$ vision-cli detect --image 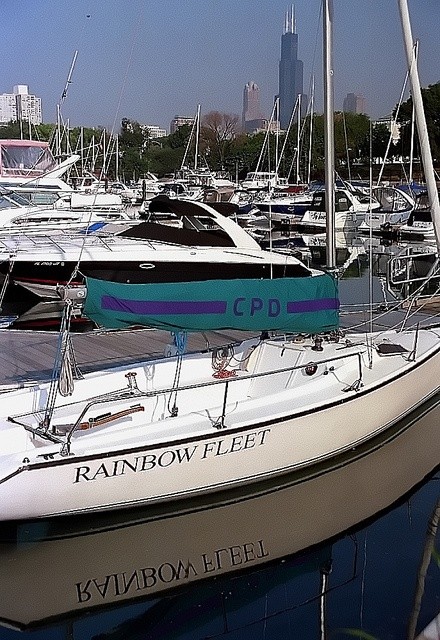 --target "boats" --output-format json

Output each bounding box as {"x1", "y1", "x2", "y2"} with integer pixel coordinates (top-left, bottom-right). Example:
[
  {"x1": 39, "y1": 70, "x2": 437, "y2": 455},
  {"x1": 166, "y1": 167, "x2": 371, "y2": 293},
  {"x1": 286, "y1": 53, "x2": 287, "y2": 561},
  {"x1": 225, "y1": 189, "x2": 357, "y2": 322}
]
[
  {"x1": 256, "y1": 190, "x2": 380, "y2": 232},
  {"x1": 0, "y1": 138, "x2": 76, "y2": 209},
  {"x1": 0, "y1": 326, "x2": 440, "y2": 640},
  {"x1": 358, "y1": 186, "x2": 419, "y2": 234},
  {"x1": 0, "y1": 194, "x2": 327, "y2": 330},
  {"x1": 259, "y1": 226, "x2": 381, "y2": 277},
  {"x1": 397, "y1": 188, "x2": 440, "y2": 257}
]
